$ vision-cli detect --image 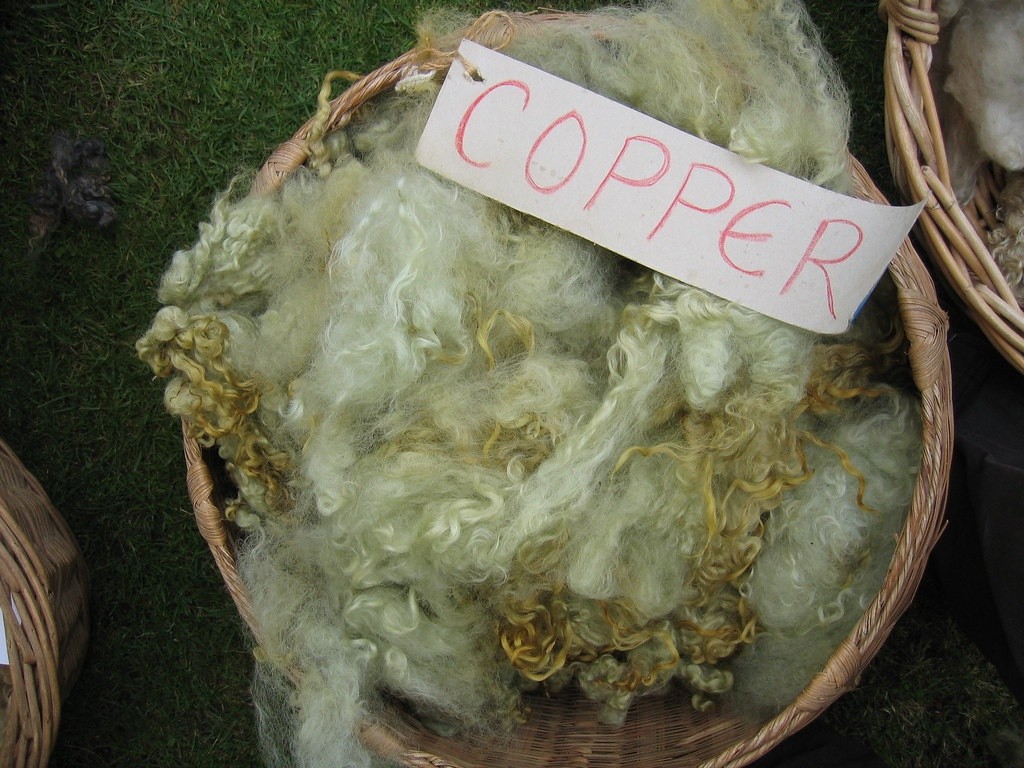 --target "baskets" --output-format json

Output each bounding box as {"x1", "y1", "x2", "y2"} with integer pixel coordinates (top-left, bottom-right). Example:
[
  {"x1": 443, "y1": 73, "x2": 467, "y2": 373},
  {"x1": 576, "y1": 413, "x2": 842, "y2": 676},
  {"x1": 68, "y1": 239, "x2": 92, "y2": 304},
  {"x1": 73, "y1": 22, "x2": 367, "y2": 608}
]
[
  {"x1": 0, "y1": 442, "x2": 87, "y2": 768},
  {"x1": 876, "y1": 2, "x2": 1023, "y2": 374},
  {"x1": 181, "y1": 13, "x2": 955, "y2": 768}
]
